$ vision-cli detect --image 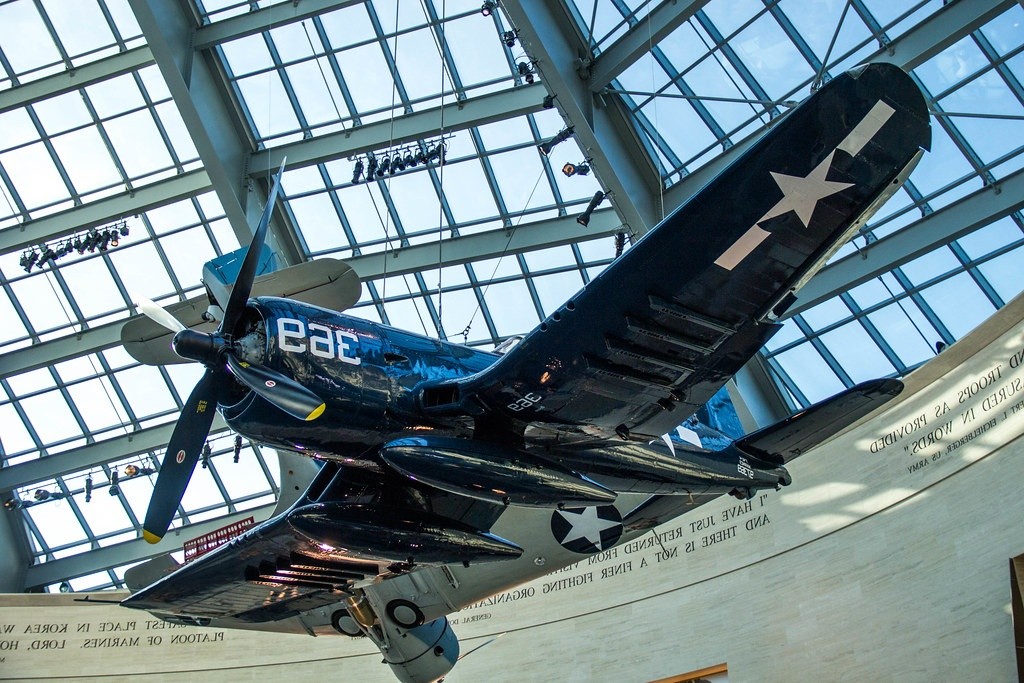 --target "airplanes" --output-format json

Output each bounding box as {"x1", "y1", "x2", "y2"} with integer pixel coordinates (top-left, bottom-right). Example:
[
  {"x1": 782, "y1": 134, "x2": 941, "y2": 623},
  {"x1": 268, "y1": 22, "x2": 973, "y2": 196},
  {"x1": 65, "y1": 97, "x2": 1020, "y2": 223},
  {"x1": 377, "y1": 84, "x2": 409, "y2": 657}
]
[{"x1": 116, "y1": 59, "x2": 936, "y2": 683}]
